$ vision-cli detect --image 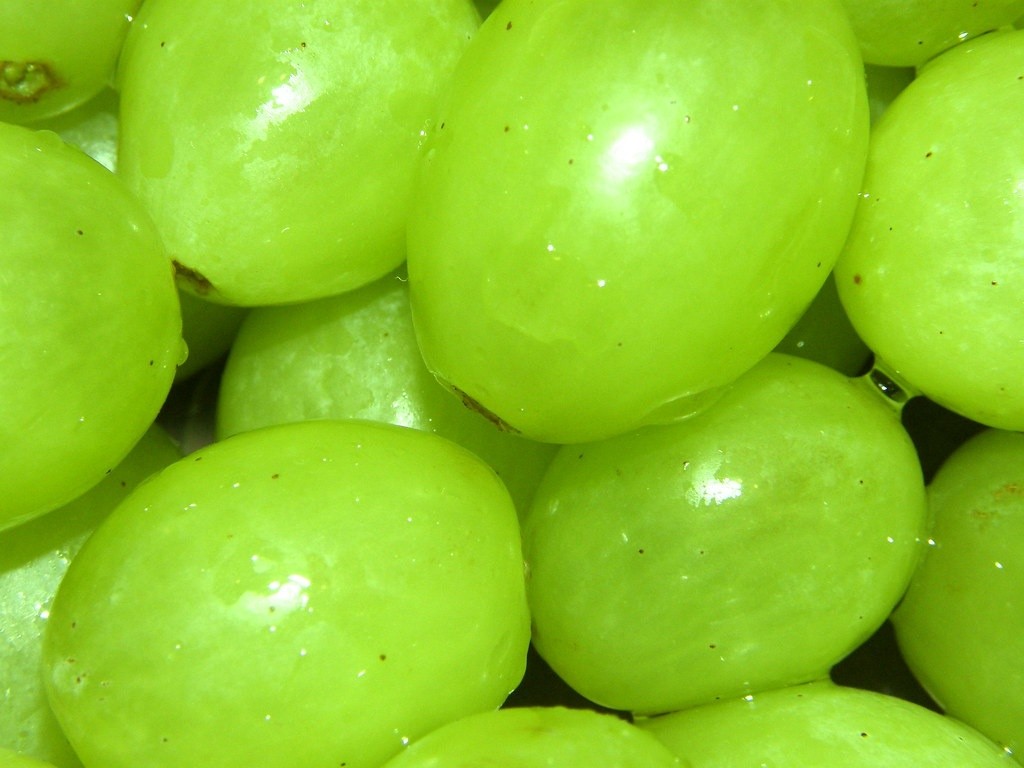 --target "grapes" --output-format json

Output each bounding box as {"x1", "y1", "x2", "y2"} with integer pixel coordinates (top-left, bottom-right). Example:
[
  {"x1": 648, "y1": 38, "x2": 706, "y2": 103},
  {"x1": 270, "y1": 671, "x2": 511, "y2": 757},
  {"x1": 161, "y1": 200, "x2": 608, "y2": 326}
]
[{"x1": 0, "y1": 0, "x2": 1024, "y2": 768}]
